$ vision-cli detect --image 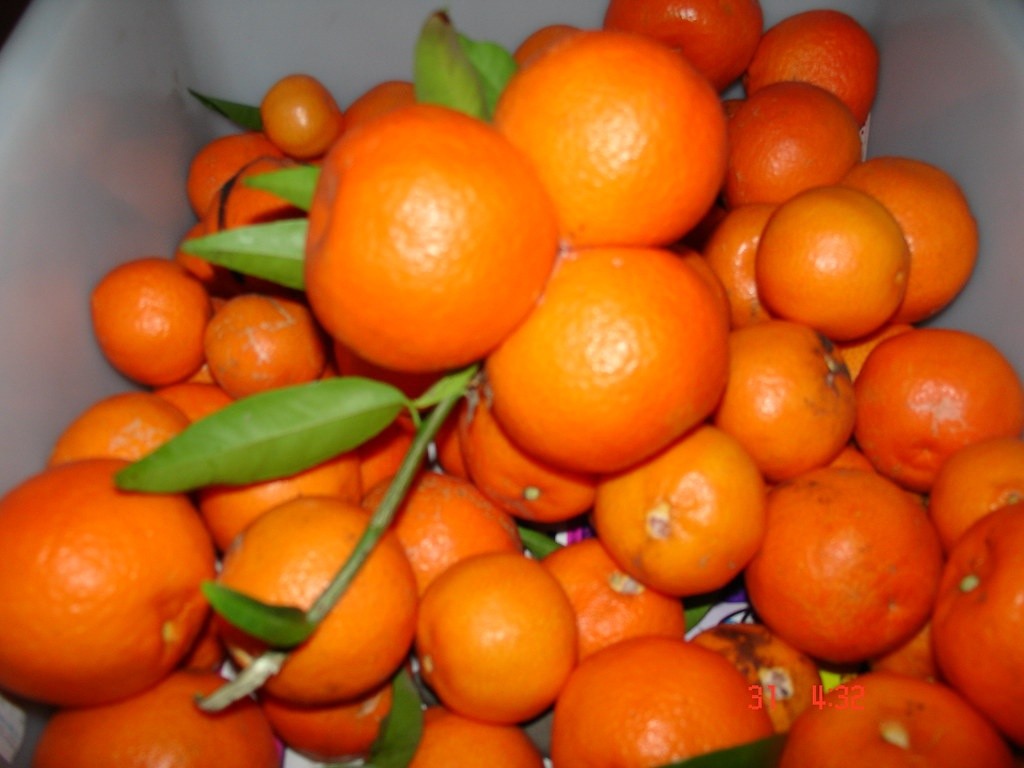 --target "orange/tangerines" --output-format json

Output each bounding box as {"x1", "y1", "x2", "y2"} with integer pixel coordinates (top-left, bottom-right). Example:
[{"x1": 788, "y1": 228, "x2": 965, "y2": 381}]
[{"x1": 1, "y1": 1, "x2": 1024, "y2": 768}]
